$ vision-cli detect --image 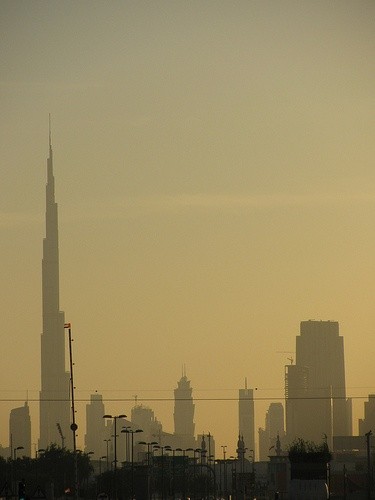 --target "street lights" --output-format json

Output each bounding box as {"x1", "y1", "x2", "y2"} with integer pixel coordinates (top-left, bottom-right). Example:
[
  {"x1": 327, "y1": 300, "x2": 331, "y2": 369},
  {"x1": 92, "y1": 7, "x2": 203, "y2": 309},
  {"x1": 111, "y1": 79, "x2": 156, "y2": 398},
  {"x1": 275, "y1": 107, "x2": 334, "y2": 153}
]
[
  {"x1": 103, "y1": 414, "x2": 127, "y2": 500},
  {"x1": 122, "y1": 426, "x2": 132, "y2": 463},
  {"x1": 121, "y1": 429, "x2": 144, "y2": 500},
  {"x1": 86, "y1": 452, "x2": 94, "y2": 488},
  {"x1": 13, "y1": 446, "x2": 23, "y2": 500},
  {"x1": 138, "y1": 440, "x2": 158, "y2": 500},
  {"x1": 64, "y1": 323, "x2": 79, "y2": 500},
  {"x1": 110, "y1": 459, "x2": 118, "y2": 483},
  {"x1": 35, "y1": 449, "x2": 45, "y2": 460},
  {"x1": 152, "y1": 445, "x2": 255, "y2": 500},
  {"x1": 104, "y1": 439, "x2": 112, "y2": 473},
  {"x1": 99, "y1": 456, "x2": 108, "y2": 478}
]
